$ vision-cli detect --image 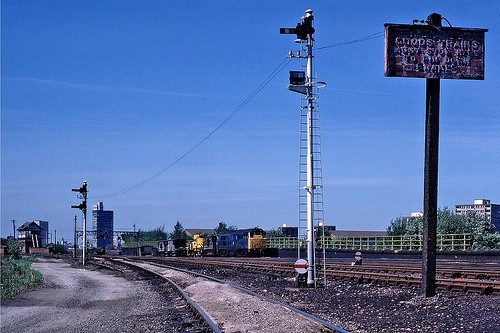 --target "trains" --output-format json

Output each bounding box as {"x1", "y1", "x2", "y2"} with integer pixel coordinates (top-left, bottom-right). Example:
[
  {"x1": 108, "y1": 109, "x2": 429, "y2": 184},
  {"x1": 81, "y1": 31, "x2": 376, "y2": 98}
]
[{"x1": 157, "y1": 228, "x2": 267, "y2": 257}]
